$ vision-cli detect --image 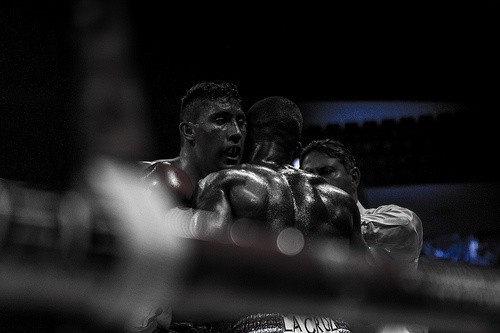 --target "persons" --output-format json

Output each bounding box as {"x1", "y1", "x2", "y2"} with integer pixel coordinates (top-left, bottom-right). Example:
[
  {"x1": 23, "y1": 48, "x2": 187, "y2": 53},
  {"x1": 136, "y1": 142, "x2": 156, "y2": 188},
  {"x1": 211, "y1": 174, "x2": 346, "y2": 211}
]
[
  {"x1": 299, "y1": 139, "x2": 432, "y2": 333},
  {"x1": 120, "y1": 80, "x2": 248, "y2": 332},
  {"x1": 172, "y1": 94, "x2": 370, "y2": 333}
]
